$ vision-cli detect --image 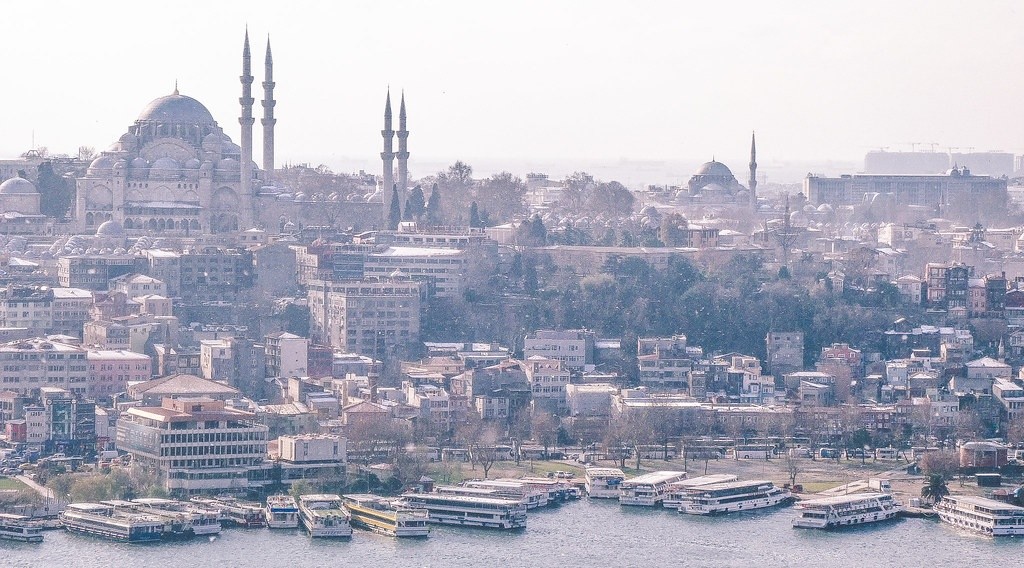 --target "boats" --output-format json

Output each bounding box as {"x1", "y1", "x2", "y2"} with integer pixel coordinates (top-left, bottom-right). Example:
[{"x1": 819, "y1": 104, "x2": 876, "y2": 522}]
[
  {"x1": 662, "y1": 473, "x2": 739, "y2": 508},
  {"x1": 61, "y1": 497, "x2": 263, "y2": 539},
  {"x1": 400, "y1": 477, "x2": 582, "y2": 530},
  {"x1": 299, "y1": 493, "x2": 354, "y2": 538},
  {"x1": 0, "y1": 514, "x2": 45, "y2": 542},
  {"x1": 585, "y1": 468, "x2": 627, "y2": 498},
  {"x1": 791, "y1": 492, "x2": 903, "y2": 529},
  {"x1": 932, "y1": 496, "x2": 1024, "y2": 536},
  {"x1": 346, "y1": 494, "x2": 430, "y2": 538},
  {"x1": 677, "y1": 479, "x2": 792, "y2": 515},
  {"x1": 265, "y1": 491, "x2": 300, "y2": 529},
  {"x1": 619, "y1": 470, "x2": 688, "y2": 506}
]
